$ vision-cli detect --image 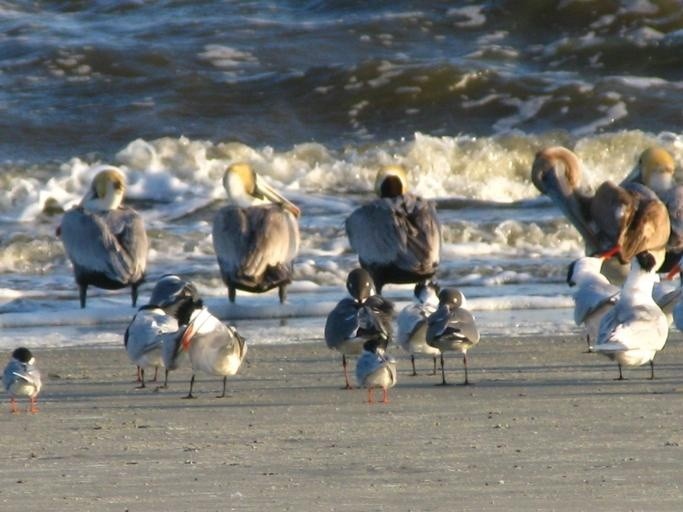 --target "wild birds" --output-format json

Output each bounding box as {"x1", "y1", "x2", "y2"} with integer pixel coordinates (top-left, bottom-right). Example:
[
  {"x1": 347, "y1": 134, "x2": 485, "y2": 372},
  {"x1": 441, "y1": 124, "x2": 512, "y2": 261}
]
[
  {"x1": 344, "y1": 163, "x2": 442, "y2": 297},
  {"x1": 212, "y1": 160, "x2": 301, "y2": 305},
  {"x1": 123, "y1": 273, "x2": 248, "y2": 399},
  {"x1": 2, "y1": 347, "x2": 42, "y2": 414},
  {"x1": 530, "y1": 145, "x2": 683, "y2": 381},
  {"x1": 324, "y1": 267, "x2": 481, "y2": 403},
  {"x1": 55, "y1": 169, "x2": 150, "y2": 308}
]
